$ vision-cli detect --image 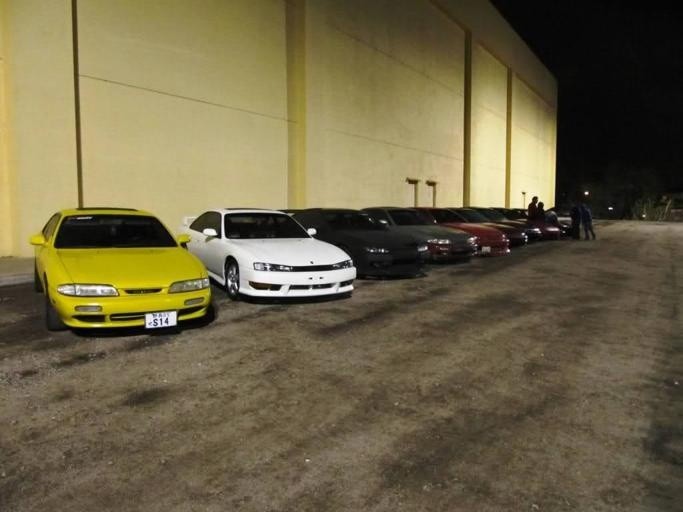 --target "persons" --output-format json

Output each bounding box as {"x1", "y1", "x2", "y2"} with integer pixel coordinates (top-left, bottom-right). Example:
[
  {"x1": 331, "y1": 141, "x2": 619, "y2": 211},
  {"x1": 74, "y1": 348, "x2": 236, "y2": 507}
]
[
  {"x1": 536, "y1": 202, "x2": 545, "y2": 226},
  {"x1": 570, "y1": 202, "x2": 581, "y2": 241},
  {"x1": 579, "y1": 202, "x2": 595, "y2": 240},
  {"x1": 528, "y1": 196, "x2": 538, "y2": 218}
]
